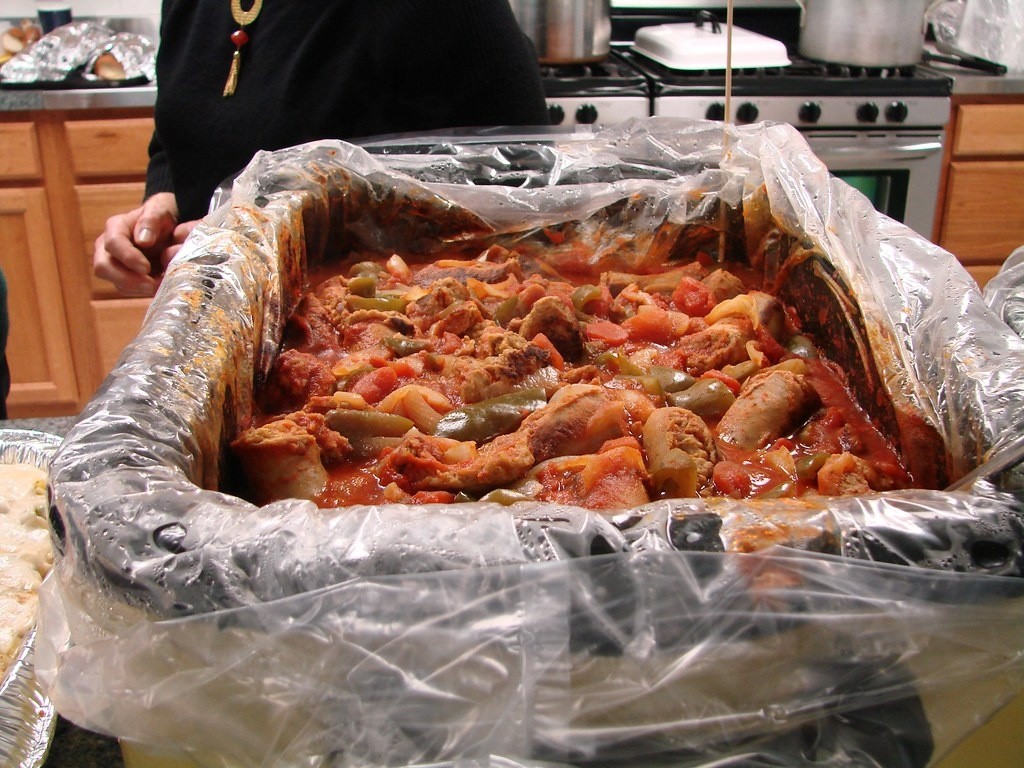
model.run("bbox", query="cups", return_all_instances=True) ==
[38,6,73,35]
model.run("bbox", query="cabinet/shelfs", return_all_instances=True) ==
[0,117,158,418]
[932,94,1024,295]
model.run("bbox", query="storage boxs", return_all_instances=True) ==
[54,142,1024,768]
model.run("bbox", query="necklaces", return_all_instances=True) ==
[223,0,262,98]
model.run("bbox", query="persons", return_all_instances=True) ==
[94,0,549,299]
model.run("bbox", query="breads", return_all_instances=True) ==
[93,51,124,80]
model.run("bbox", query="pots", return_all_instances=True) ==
[796,0,940,66]
[510,0,614,63]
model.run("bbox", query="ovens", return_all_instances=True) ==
[795,128,949,257]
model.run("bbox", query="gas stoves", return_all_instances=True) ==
[540,42,952,128]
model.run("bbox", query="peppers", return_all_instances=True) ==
[325,263,828,505]
